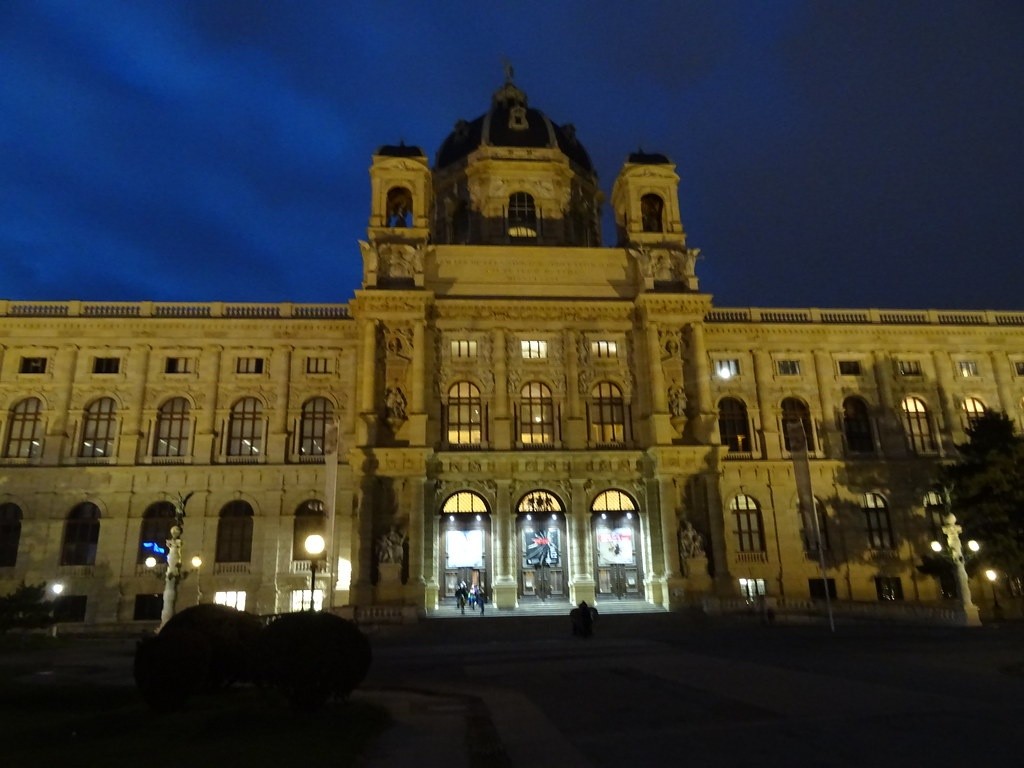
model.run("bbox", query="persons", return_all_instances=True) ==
[679,520,706,559]
[454,581,484,616]
[667,374,688,416]
[384,387,404,418]
[375,526,407,564]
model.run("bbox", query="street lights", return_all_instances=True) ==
[927,512,982,624]
[302,532,327,612]
[143,526,204,637]
